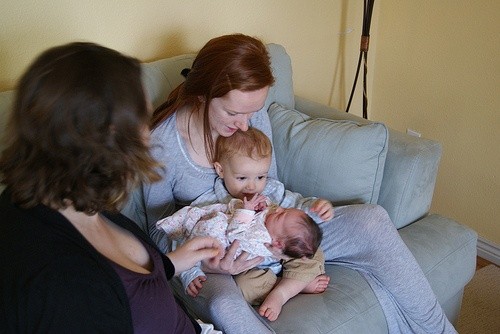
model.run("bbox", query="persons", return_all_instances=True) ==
[115,34,457,334]
[171,125,334,323]
[156,193,323,259]
[0,41,225,333]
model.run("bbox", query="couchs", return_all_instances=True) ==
[0,44,477,334]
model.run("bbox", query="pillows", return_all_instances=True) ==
[267,103,390,205]
